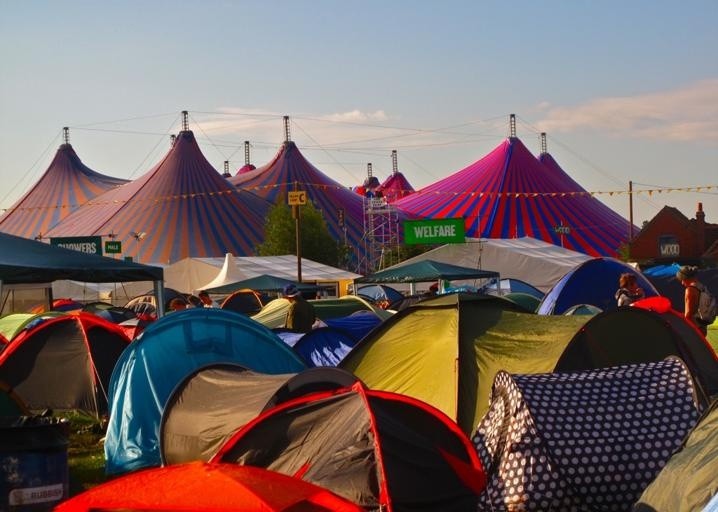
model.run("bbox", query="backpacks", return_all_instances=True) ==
[692,284,718,324]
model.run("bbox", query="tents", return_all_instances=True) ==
[0,235,718,512]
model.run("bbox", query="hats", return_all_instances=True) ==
[284,284,300,298]
[677,266,698,279]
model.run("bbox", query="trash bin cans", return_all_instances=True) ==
[0,415,69,512]
